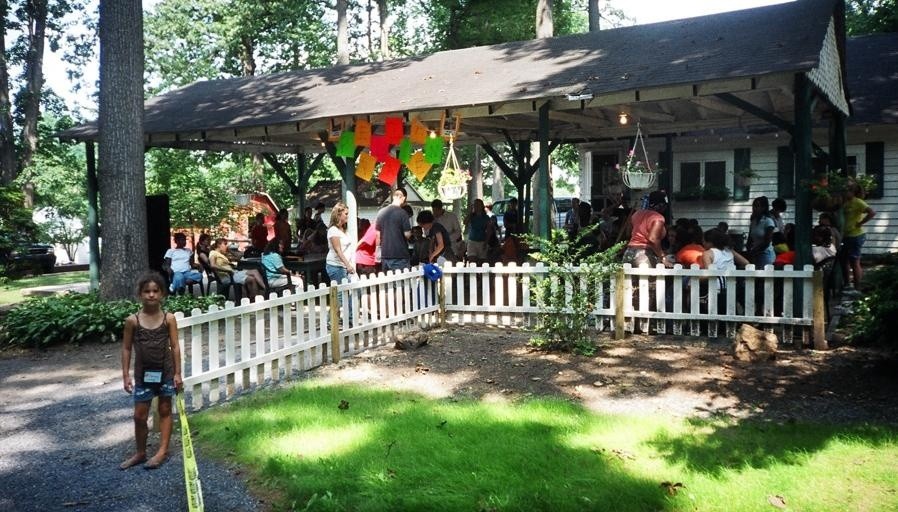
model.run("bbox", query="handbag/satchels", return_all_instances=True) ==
[143,369,163,384]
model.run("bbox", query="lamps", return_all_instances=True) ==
[619,111,628,125]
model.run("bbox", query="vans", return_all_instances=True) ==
[491,197,573,233]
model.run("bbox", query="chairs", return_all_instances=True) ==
[256,260,307,310]
[166,261,205,298]
[209,266,243,300]
[197,256,217,296]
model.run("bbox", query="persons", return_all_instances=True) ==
[120,272,182,470]
[840,183,875,290]
[563,197,842,334]
[162,188,524,315]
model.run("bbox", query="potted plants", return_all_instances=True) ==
[675,184,701,200]
[702,185,731,199]
[11,207,56,274]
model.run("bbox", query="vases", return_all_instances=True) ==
[819,198,834,209]
[735,188,743,200]
[625,173,653,189]
[855,192,868,199]
[443,185,463,197]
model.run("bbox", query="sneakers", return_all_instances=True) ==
[168,283,175,294]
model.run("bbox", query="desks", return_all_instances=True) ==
[238,253,331,305]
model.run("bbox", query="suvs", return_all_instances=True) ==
[0,233,56,274]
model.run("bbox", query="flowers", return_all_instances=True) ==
[436,168,473,185]
[729,167,761,187]
[615,149,664,176]
[843,172,879,194]
[798,169,848,197]
